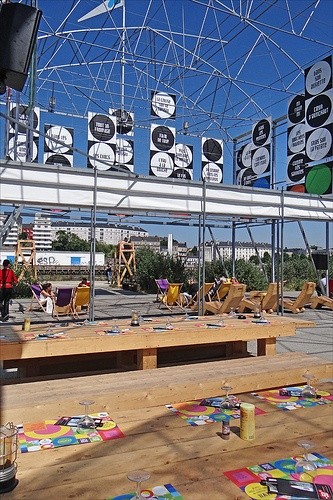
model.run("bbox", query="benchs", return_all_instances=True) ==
[0,352,333,429]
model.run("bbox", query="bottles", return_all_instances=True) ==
[222,420,230,440]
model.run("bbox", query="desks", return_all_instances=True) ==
[0,313,296,370]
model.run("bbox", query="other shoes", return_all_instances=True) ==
[0,317,9,321]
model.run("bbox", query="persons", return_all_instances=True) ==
[230,277,240,285]
[0,259,17,321]
[78,278,90,311]
[38,283,58,316]
[316,273,327,297]
[201,274,227,302]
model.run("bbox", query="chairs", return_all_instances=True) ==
[156,279,333,314]
[27,283,90,321]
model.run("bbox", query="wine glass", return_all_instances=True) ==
[300,374,318,401]
[184,307,266,326]
[138,312,144,323]
[76,399,96,435]
[294,439,317,482]
[46,323,53,337]
[166,317,172,329]
[111,319,120,333]
[126,470,152,500]
[83,316,90,327]
[220,387,233,415]
